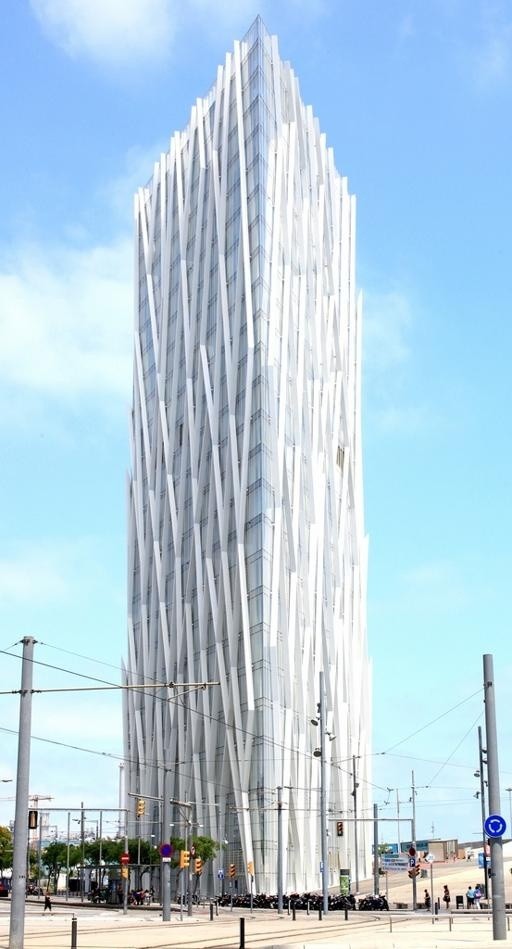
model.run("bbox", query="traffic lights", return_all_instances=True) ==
[229,863,235,877]
[195,858,202,876]
[180,850,190,869]
[137,800,145,817]
[337,822,343,836]
[247,862,253,873]
[121,868,128,879]
[408,864,420,879]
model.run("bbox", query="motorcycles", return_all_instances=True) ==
[213,892,390,911]
[176,892,201,905]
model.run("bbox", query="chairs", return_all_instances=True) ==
[456,896,464,909]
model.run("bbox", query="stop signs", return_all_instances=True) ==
[119,853,130,864]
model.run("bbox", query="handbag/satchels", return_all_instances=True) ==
[475,893,481,897]
[444,896,450,901]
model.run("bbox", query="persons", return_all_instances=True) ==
[131,885,154,905]
[466,886,474,909]
[444,885,450,908]
[424,889,431,911]
[474,886,481,909]
[42,892,54,916]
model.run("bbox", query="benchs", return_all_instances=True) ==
[416,903,427,909]
[393,903,409,909]
